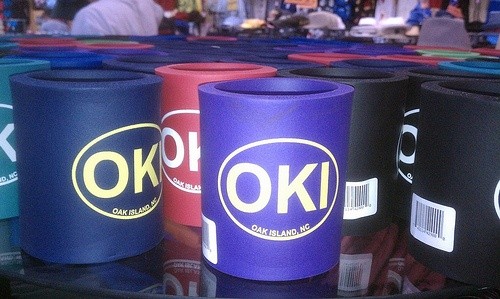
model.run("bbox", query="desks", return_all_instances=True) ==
[0,214,498,299]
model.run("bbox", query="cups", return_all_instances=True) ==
[0,33,499,283]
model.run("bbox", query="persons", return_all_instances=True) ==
[41,1,90,34]
[406,0,432,25]
[73,0,164,36]
